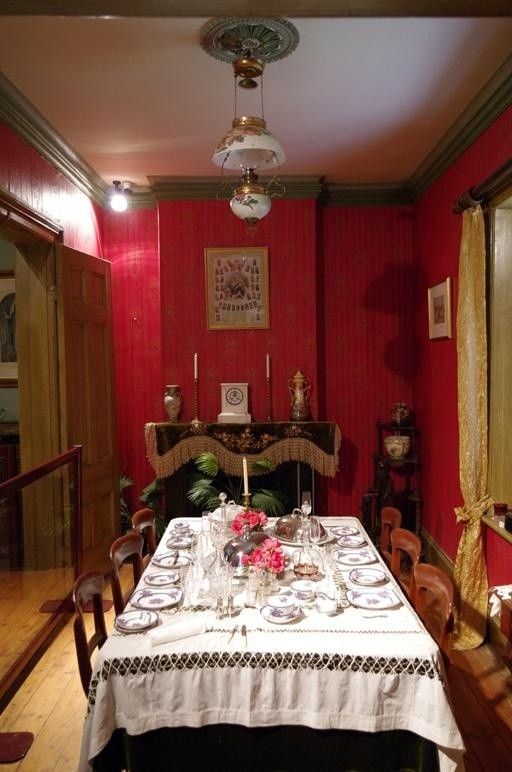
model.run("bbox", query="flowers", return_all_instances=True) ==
[277,526,290,541]
[231,511,268,535]
[239,538,289,578]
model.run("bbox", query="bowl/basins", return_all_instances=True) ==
[212,519,233,528]
[386,436,410,460]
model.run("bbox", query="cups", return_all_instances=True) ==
[266,595,295,617]
[201,511,212,532]
[291,580,316,602]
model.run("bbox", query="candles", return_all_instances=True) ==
[242,456,250,494]
[193,352,199,379]
[266,353,271,378]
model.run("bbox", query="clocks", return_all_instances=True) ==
[217,382,253,424]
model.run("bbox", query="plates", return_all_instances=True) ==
[265,525,334,545]
[259,606,305,623]
[330,525,400,611]
[116,521,205,632]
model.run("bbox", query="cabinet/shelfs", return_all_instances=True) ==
[362,417,424,559]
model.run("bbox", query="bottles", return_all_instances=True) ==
[292,526,324,579]
[228,594,235,617]
[216,594,224,619]
[206,544,234,592]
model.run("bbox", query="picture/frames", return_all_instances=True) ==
[427,276,454,341]
[0,269,19,388]
[203,245,273,331]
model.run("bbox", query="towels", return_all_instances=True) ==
[148,613,207,647]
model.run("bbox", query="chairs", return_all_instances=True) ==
[106,531,144,615]
[407,562,456,676]
[131,507,157,562]
[379,507,402,566]
[69,570,109,699]
[388,527,422,602]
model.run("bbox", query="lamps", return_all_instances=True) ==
[198,16,302,239]
